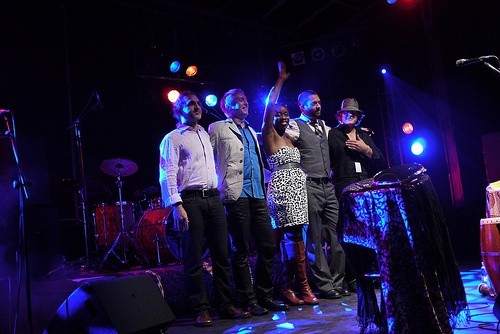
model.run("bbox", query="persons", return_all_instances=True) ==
[261,60,317,304]
[160,88,388,324]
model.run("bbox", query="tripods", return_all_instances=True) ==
[97,170,153,274]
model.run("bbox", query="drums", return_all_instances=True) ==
[93,199,137,250]
[479,217,500,294]
[144,196,165,209]
[134,209,211,263]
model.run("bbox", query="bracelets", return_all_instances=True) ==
[174,202,181,206]
[366,146,371,154]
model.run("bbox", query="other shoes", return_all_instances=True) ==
[349,279,357,292]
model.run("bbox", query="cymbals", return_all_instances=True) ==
[100,158,138,177]
[121,185,161,201]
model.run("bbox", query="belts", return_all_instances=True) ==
[306,177,331,182]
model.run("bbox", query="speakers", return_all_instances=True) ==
[42,273,176,334]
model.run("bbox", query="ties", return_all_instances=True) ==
[314,123,324,140]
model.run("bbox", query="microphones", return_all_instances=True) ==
[96,93,102,111]
[456,56,497,66]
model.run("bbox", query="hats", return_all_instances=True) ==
[337,97,363,115]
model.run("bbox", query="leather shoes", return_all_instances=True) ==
[265,302,289,312]
[320,288,351,299]
[195,310,213,327]
[243,304,269,316]
[181,188,221,197]
[221,306,251,320]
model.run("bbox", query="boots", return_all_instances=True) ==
[279,240,305,306]
[293,239,320,305]
